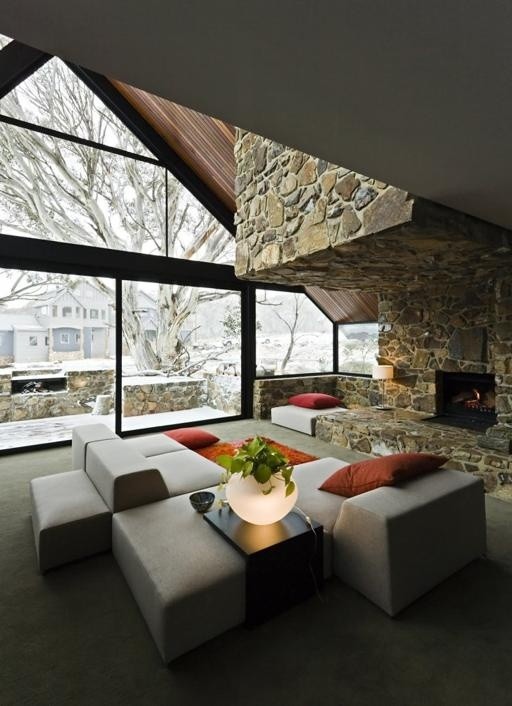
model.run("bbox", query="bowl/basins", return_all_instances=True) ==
[189,491,215,513]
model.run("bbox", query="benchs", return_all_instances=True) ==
[112,458,485,666]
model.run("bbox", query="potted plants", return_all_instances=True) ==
[223,437,297,524]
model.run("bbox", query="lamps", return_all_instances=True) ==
[373,366,394,411]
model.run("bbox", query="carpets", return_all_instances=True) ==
[191,436,318,466]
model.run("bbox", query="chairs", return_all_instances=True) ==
[29,425,232,577]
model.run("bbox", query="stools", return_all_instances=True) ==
[270,405,349,437]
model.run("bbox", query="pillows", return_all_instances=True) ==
[319,453,451,497]
[287,393,342,409]
